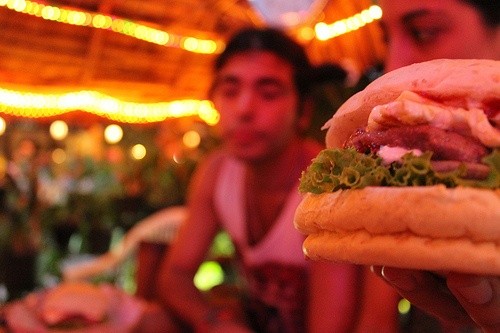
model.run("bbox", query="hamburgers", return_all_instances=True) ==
[290,58,499,278]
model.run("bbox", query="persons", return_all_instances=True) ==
[0,122,221,301]
[305,57,384,147]
[153,25,364,330]
[379,0,499,331]
